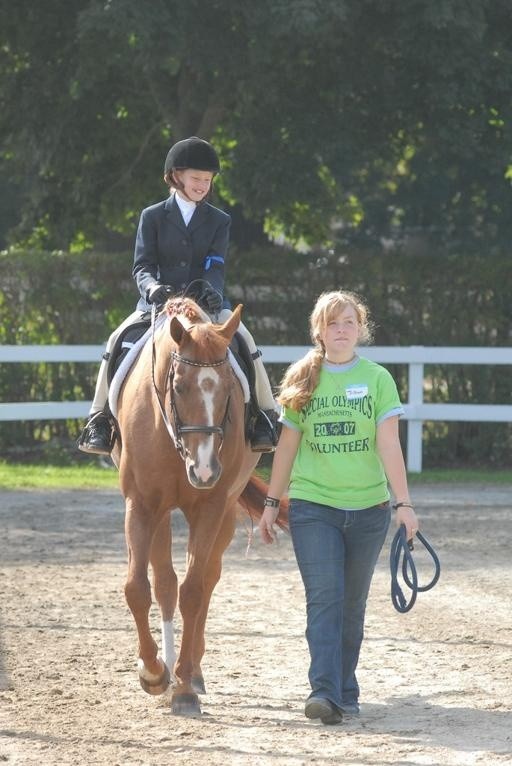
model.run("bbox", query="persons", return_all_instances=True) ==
[257,288,419,726]
[76,135,282,456]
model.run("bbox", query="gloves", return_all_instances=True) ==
[204,287,224,313]
[146,283,173,306]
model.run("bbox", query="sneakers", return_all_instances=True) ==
[75,412,112,455]
[304,695,344,726]
[249,409,282,453]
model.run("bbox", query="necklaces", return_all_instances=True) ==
[323,352,357,365]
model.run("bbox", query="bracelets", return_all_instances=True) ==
[263,495,280,509]
[393,501,414,510]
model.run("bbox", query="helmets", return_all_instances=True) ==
[163,136,222,179]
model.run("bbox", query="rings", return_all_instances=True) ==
[410,527,416,534]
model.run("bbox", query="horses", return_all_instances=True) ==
[108,296,292,722]
